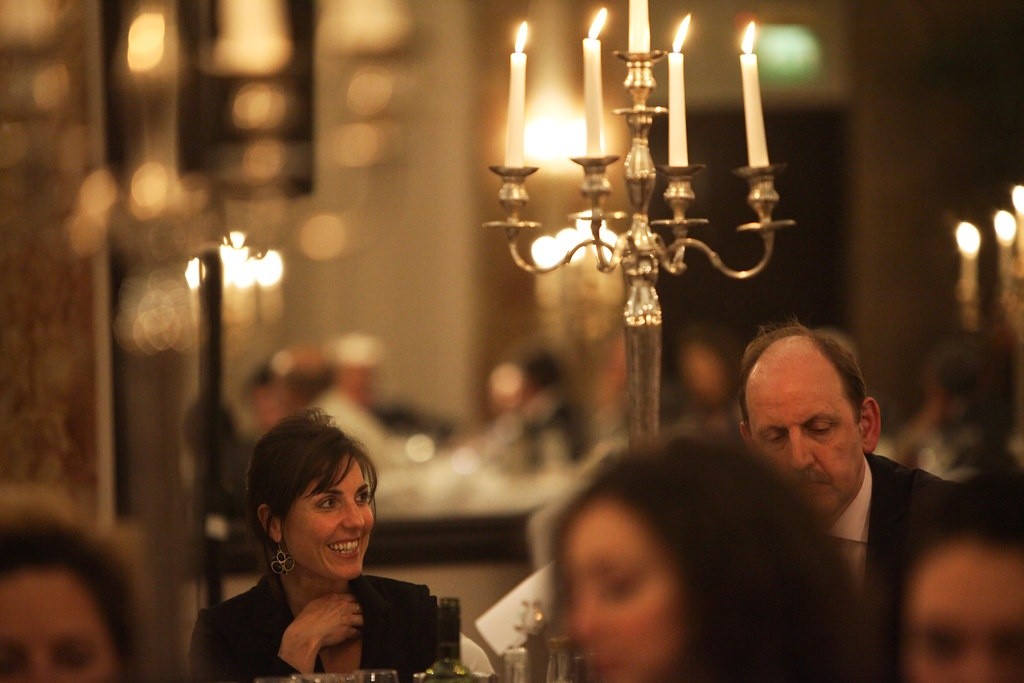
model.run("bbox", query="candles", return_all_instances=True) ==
[739,20,769,166]
[581,7,611,157]
[953,219,983,335]
[667,13,693,167]
[628,0,650,55]
[501,21,530,169]
[992,208,1018,321]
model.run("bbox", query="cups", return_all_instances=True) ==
[254,669,398,683]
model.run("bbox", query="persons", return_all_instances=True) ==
[541,428,860,683]
[872,467,1023,683]
[181,408,447,683]
[738,323,970,597]
[222,321,1024,488]
[0,475,162,683]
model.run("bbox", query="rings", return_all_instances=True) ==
[352,601,362,615]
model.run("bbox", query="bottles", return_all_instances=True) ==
[502,646,532,683]
[545,636,574,683]
[422,598,479,683]
